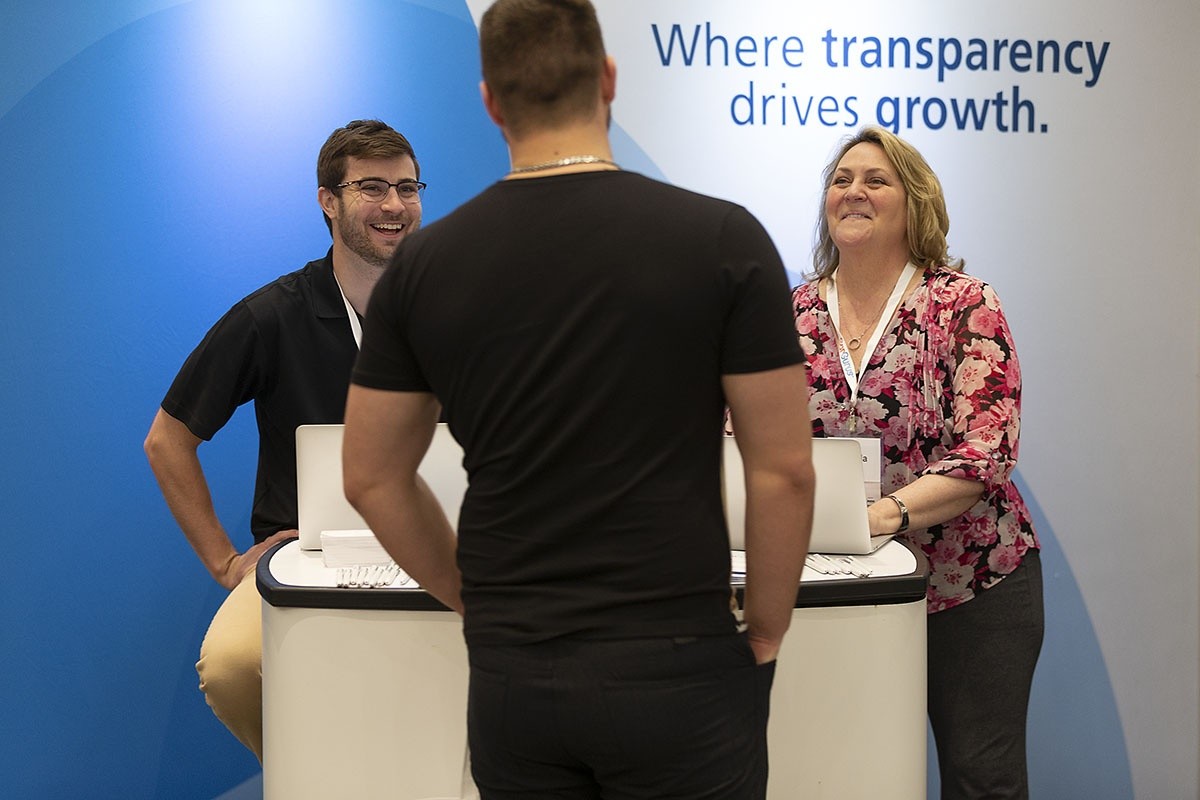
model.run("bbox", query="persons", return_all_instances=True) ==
[141,121,428,774]
[784,127,1044,800]
[339,1,792,800]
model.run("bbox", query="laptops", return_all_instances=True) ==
[295,424,469,551]
[722,435,893,554]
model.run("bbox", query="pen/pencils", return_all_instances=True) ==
[841,555,873,579]
[335,560,411,588]
[804,554,851,574]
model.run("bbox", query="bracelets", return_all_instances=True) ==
[883,493,910,535]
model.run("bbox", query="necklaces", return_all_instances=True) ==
[507,156,620,175]
[838,257,909,350]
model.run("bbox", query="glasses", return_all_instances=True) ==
[336,178,426,204]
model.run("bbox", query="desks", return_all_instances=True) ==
[254,535,930,799]
